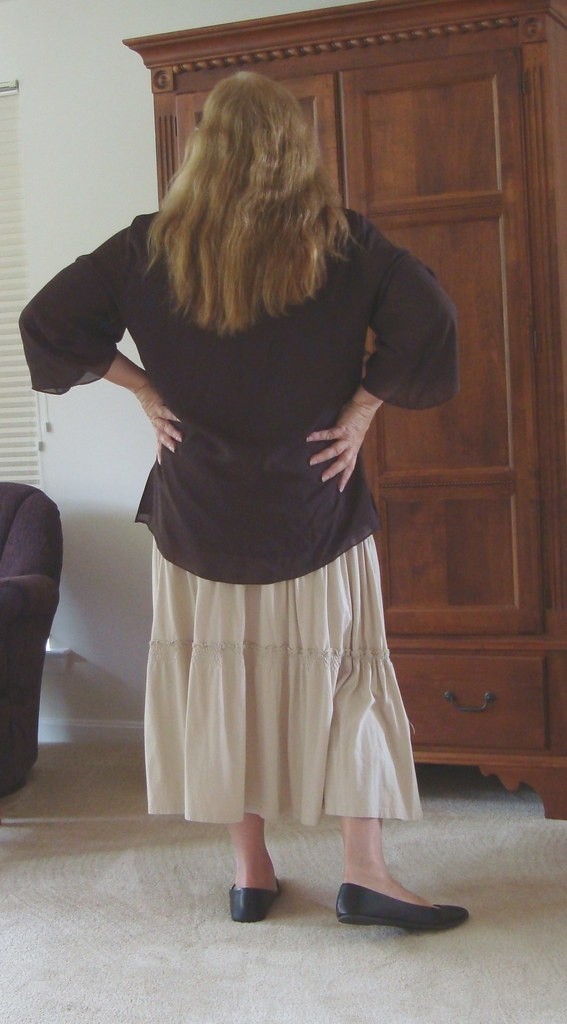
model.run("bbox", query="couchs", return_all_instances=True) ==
[0,481,65,797]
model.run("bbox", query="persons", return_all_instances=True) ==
[20,70,470,935]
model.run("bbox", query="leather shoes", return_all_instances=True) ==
[336,883,468,929]
[230,876,281,923]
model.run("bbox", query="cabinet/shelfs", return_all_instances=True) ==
[121,0,567,821]
